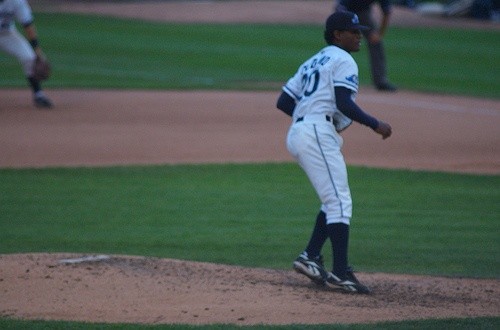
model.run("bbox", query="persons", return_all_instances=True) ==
[275,9,391,292]
[332,0,398,92]
[0,0,53,108]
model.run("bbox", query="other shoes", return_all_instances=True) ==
[378,81,397,91]
[33,95,53,109]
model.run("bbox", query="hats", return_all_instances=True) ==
[325,10,372,32]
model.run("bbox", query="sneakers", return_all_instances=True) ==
[320,267,370,294]
[293,251,328,281]
